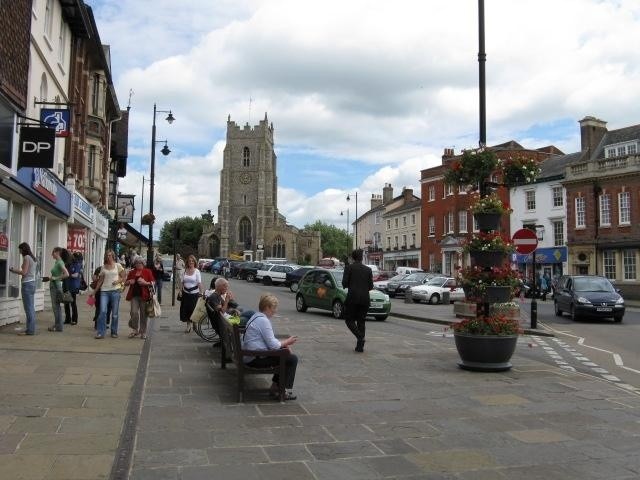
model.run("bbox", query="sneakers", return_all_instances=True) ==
[95,334,104,339]
[140,332,146,339]
[17,329,34,335]
[111,334,118,338]
[48,325,64,332]
[127,329,139,338]
[355,340,365,352]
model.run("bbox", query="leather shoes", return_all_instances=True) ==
[269,388,297,400]
[287,391,292,394]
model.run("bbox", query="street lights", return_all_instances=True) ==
[340,209,349,256]
[346,192,358,250]
[146,102,176,268]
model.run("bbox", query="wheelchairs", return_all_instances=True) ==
[191,294,220,342]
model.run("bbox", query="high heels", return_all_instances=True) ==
[64,317,71,324]
[71,314,78,325]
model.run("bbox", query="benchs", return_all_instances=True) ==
[204,302,246,348]
[219,311,291,403]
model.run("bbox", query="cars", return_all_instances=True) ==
[160,258,174,280]
[409,277,466,304]
[285,266,323,294]
[197,256,296,284]
[293,263,391,322]
[373,273,403,291]
[554,275,625,322]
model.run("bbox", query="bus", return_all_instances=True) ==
[319,258,340,268]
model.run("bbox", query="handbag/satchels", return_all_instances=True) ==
[55,289,74,304]
[78,277,88,291]
[177,293,183,301]
[7,284,19,298]
[146,297,155,318]
[119,282,125,293]
[152,294,162,319]
[90,281,97,289]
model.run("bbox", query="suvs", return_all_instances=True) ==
[387,272,440,297]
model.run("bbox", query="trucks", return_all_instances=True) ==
[396,267,425,275]
[366,264,378,272]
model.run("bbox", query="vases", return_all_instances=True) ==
[463,286,477,299]
[486,286,512,305]
[476,214,500,230]
[474,252,504,267]
[508,169,526,183]
[454,335,519,371]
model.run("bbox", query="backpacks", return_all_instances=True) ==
[190,296,208,325]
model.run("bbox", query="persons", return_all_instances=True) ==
[63,248,82,326]
[513,268,559,301]
[175,253,184,290]
[240,291,299,401]
[341,249,374,352]
[203,276,218,299]
[94,248,126,338]
[9,241,39,337]
[178,254,203,334]
[47,246,69,332]
[91,265,112,330]
[220,258,231,275]
[125,256,157,340]
[207,278,242,316]
[153,252,164,306]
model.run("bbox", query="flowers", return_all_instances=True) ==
[444,141,501,190]
[486,264,524,287]
[467,192,513,216]
[458,231,518,255]
[501,156,542,185]
[444,314,524,336]
[454,265,485,303]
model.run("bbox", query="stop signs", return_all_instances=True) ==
[511,228,538,255]
